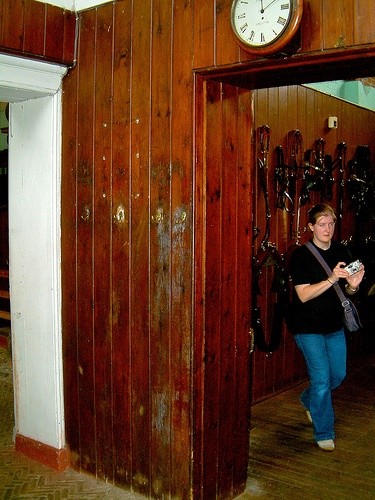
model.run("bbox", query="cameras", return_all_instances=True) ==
[344,259,361,276]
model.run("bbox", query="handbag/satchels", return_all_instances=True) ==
[342,299,362,332]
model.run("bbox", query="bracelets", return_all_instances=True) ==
[346,285,359,295]
[326,279,334,285]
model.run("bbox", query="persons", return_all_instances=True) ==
[292,203,365,452]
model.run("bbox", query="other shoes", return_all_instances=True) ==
[306,408,312,422]
[318,439,334,451]
